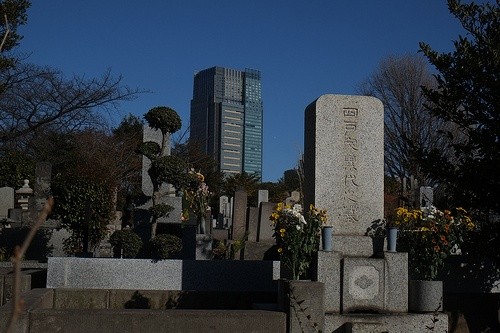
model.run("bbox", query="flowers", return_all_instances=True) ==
[385,207,476,281]
[271,202,329,280]
[179,167,213,221]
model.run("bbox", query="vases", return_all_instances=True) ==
[200,216,206,234]
[409,280,444,312]
